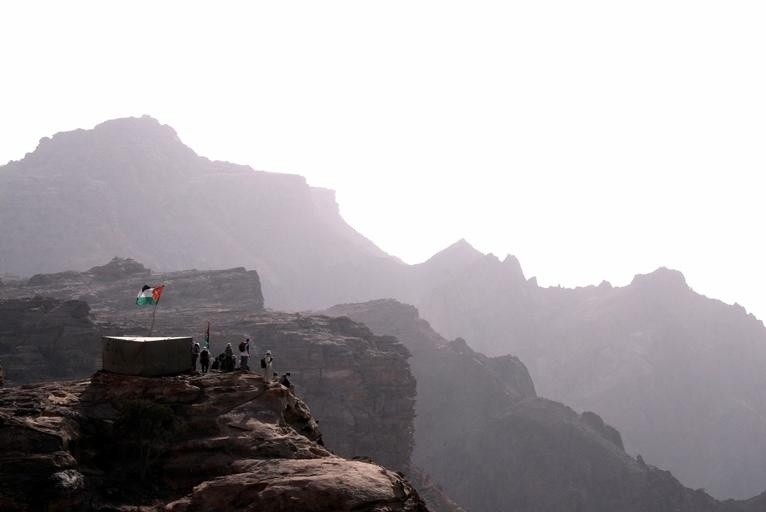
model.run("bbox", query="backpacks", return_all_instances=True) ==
[239,341,247,352]
[260,358,267,368]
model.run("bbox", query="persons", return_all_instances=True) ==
[261,350,273,384]
[279,370,295,394]
[192,339,252,373]
[273,372,282,385]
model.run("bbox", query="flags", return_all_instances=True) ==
[134,285,163,306]
[203,323,210,348]
